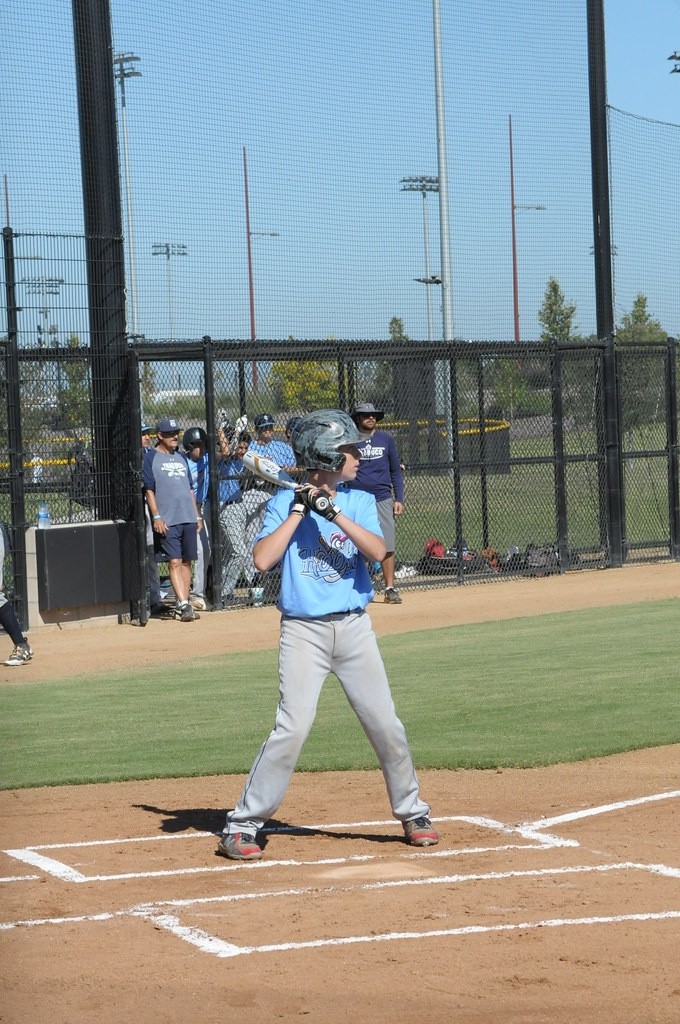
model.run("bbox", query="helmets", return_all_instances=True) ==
[285,416,301,430]
[291,409,375,473]
[229,431,252,444]
[254,414,275,427]
[216,426,235,444]
[183,427,206,451]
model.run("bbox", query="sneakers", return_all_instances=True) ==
[402,817,438,846]
[173,602,200,622]
[218,832,263,860]
[4,636,33,666]
[384,587,402,604]
[394,565,418,579]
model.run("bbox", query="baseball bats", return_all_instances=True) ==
[242,450,329,512]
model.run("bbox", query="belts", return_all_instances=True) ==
[225,499,243,505]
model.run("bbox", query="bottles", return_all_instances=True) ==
[38,504,50,529]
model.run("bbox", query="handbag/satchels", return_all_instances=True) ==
[415,539,580,574]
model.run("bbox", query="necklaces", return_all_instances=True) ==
[356,434,374,462]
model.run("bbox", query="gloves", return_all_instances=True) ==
[300,486,342,523]
[291,484,310,519]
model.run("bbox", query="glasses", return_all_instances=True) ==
[163,430,181,434]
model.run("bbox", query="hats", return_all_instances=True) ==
[348,403,385,422]
[141,421,155,432]
[156,418,185,432]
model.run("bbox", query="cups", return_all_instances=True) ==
[252,588,264,606]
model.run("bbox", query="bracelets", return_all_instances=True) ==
[153,515,160,522]
[197,517,202,520]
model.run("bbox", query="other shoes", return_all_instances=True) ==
[151,604,174,618]
[189,595,213,610]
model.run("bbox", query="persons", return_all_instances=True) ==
[216,414,308,610]
[30,453,43,484]
[180,408,230,610]
[0,523,34,666]
[140,418,171,618]
[143,419,203,622]
[218,408,440,861]
[346,403,404,604]
